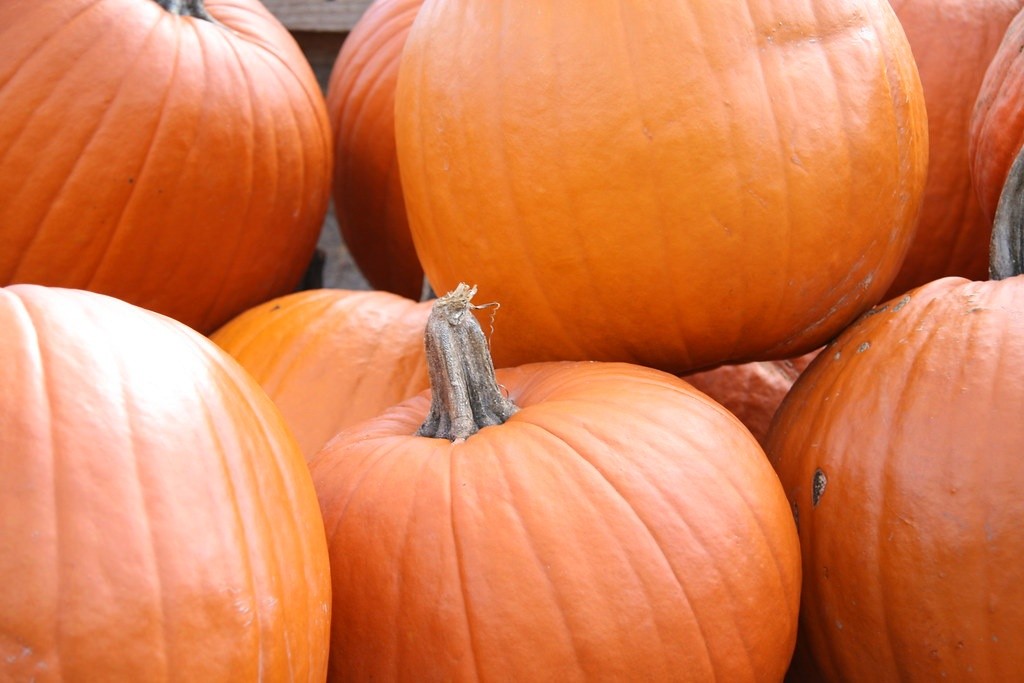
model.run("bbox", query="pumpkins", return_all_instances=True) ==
[0,0,1024,683]
